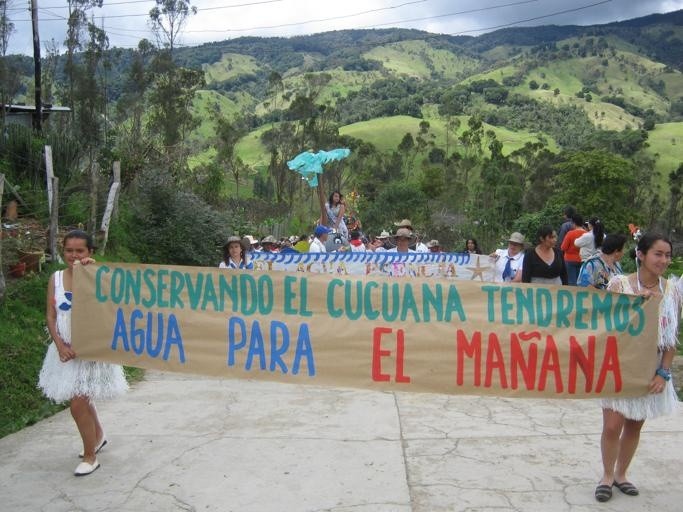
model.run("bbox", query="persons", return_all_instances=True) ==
[561,214,588,285]
[575,217,610,267]
[490,231,526,284]
[596,234,680,500]
[324,191,349,239]
[460,238,483,254]
[521,225,571,285]
[218,236,252,271]
[37,231,130,476]
[577,230,629,292]
[244,217,445,253]
[558,206,576,268]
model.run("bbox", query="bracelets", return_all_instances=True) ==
[658,369,670,379]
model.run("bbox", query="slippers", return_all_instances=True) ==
[595,478,615,501]
[615,480,638,495]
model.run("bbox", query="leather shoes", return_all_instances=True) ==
[74,457,100,476]
[78,435,107,458]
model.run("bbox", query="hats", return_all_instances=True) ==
[224,235,280,253]
[315,219,440,248]
[505,232,533,250]
